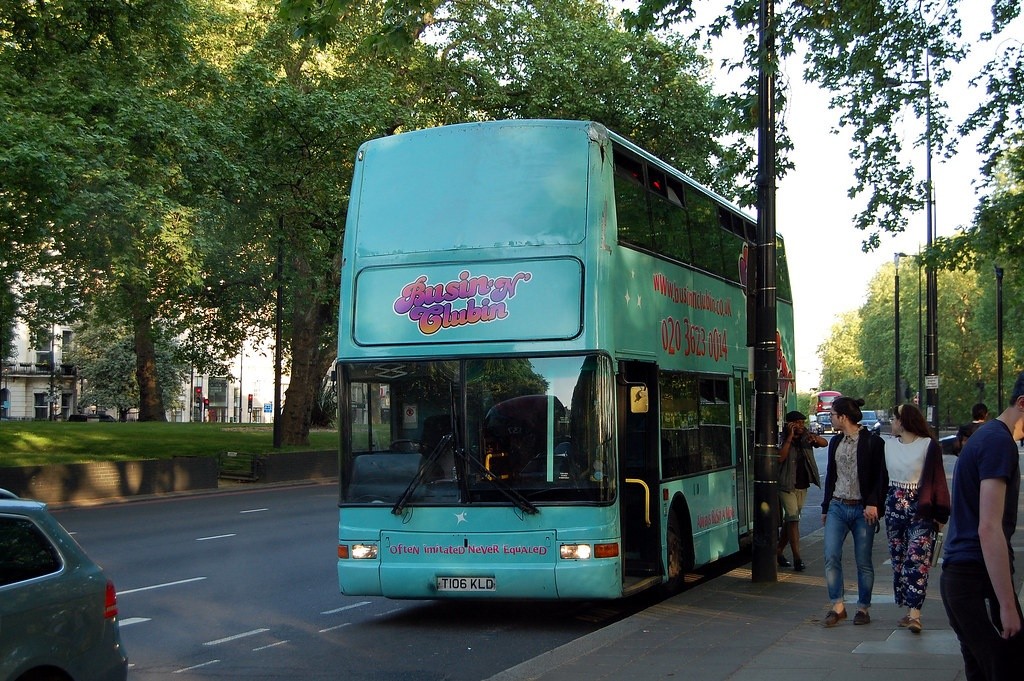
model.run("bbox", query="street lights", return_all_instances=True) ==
[992,258,1003,416]
[892,252,909,408]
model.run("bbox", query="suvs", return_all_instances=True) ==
[0,488,129,681]
[816,411,839,435]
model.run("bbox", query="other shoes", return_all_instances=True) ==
[794,559,805,570]
[777,555,791,567]
[908,618,922,633]
[854,610,870,624]
[897,615,909,627]
[821,607,848,625]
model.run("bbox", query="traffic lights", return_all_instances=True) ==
[194,387,202,403]
[248,394,253,413]
[205,399,209,409]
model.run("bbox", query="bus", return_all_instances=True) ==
[337,119,798,601]
[808,390,842,433]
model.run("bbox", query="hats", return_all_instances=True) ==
[786,410,806,421]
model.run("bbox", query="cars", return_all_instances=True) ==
[90,414,115,422]
[857,410,881,435]
[808,415,821,435]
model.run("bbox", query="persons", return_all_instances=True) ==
[939,372,1024,681]
[821,397,886,627]
[956,403,988,453]
[863,405,949,633]
[779,411,828,570]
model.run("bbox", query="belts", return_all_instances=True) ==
[833,496,857,505]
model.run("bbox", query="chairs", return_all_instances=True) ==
[419,415,463,459]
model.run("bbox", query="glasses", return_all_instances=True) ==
[829,413,841,418]
[889,416,897,424]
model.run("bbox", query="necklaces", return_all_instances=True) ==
[912,434,917,442]
